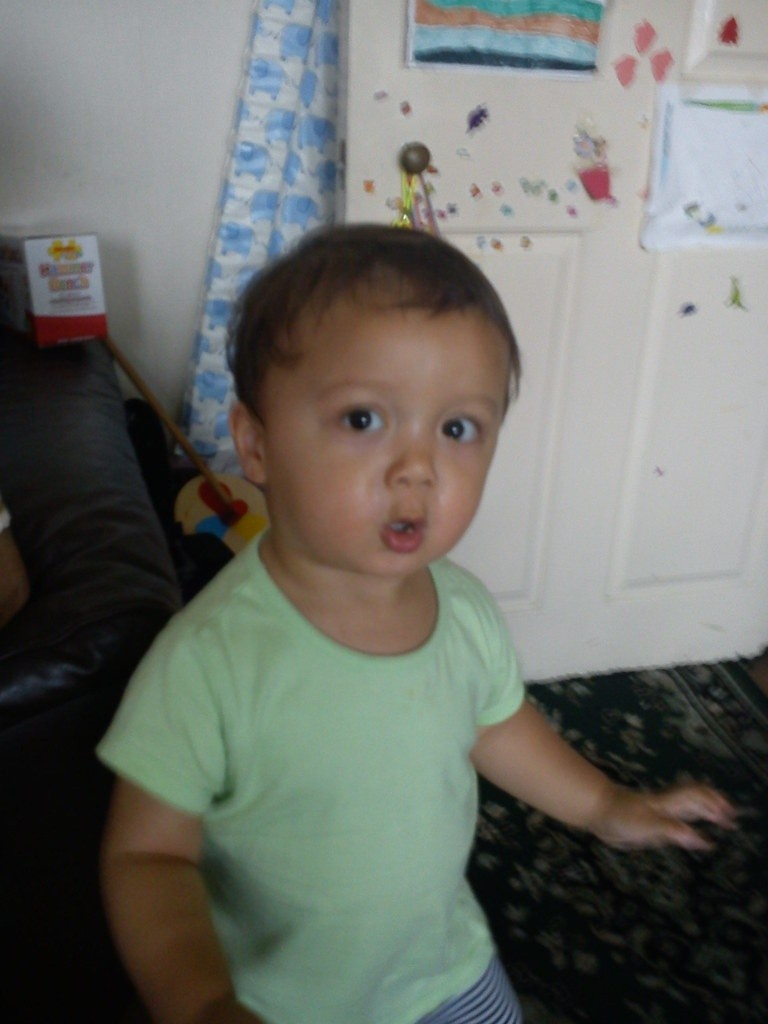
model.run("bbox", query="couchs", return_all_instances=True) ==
[0,328,193,1024]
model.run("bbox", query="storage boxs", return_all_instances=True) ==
[23,236,110,346]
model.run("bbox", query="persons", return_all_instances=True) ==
[97,222,737,1023]
